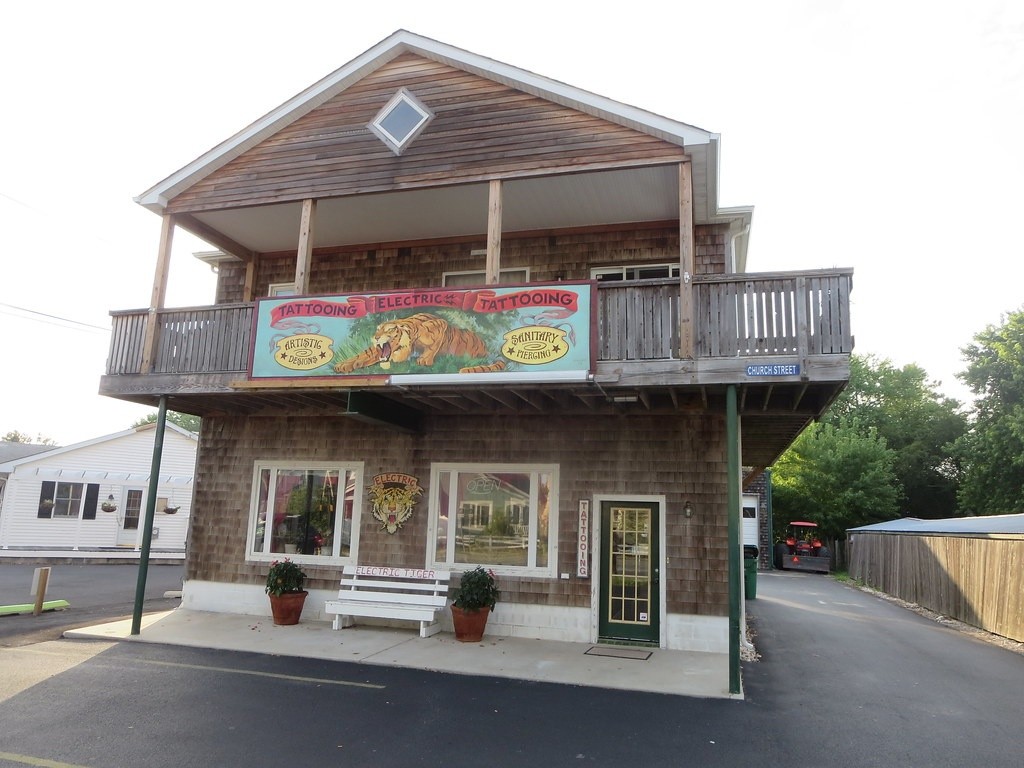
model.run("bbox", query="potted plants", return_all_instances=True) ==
[39,498,54,510]
[101,502,116,512]
[163,506,181,514]
[264,557,308,625]
[449,565,502,642]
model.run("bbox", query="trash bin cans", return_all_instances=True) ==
[744,543,760,599]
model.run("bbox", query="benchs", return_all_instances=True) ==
[324,565,451,638]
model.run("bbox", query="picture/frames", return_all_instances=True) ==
[246,279,598,380]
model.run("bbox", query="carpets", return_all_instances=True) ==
[583,646,653,660]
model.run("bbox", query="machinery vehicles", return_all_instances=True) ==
[774,522,831,575]
